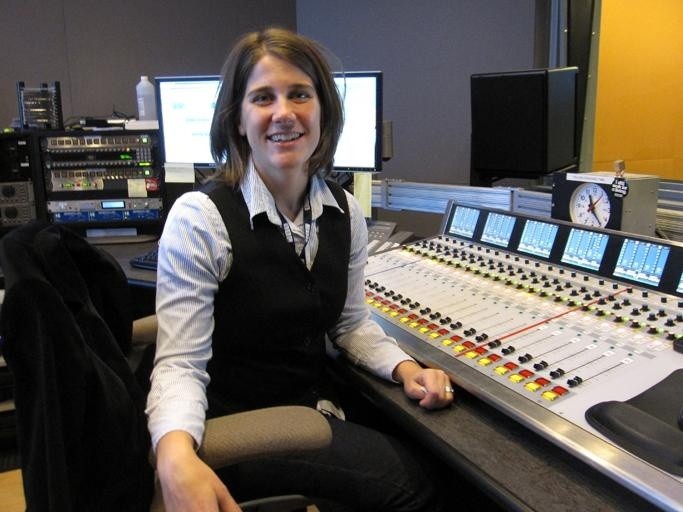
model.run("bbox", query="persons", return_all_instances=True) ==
[141,24,456,511]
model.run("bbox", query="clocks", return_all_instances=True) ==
[550,171,658,238]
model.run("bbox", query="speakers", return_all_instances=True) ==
[469,65,580,186]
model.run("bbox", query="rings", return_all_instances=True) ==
[446,387,455,393]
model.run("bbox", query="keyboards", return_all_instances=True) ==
[129,247,158,270]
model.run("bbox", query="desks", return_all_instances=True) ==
[93,238,683,512]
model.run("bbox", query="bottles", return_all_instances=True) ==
[137,76,157,121]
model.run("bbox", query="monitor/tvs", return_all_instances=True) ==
[153,70,386,176]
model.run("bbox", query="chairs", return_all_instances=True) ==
[1,217,334,512]
